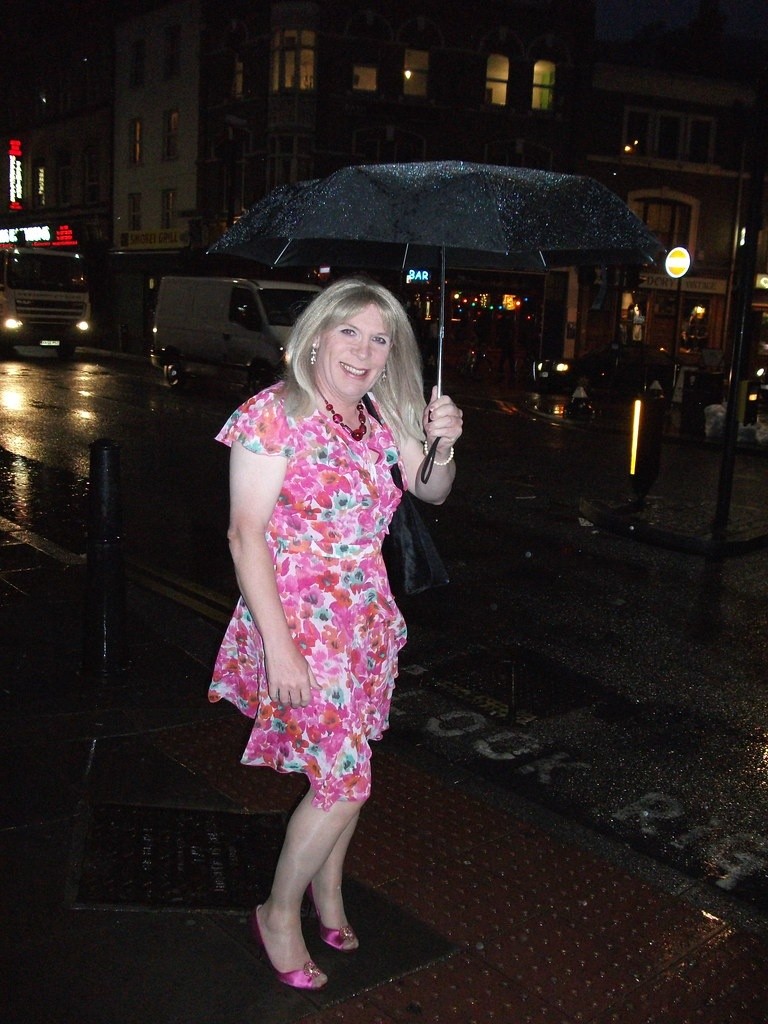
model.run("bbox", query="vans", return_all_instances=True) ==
[150,275,327,390]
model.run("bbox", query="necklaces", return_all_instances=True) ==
[311,379,367,442]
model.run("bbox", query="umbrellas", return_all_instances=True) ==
[202,164,663,489]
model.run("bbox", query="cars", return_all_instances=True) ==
[532,345,662,393]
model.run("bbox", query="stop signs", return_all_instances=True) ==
[664,247,691,279]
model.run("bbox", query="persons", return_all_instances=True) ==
[204,277,464,992]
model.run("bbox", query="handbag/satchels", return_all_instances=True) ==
[362,394,450,604]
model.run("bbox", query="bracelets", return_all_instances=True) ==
[423,441,455,466]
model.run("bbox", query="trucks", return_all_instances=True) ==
[0,246,91,352]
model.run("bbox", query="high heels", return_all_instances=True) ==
[248,906,328,991]
[301,882,360,954]
